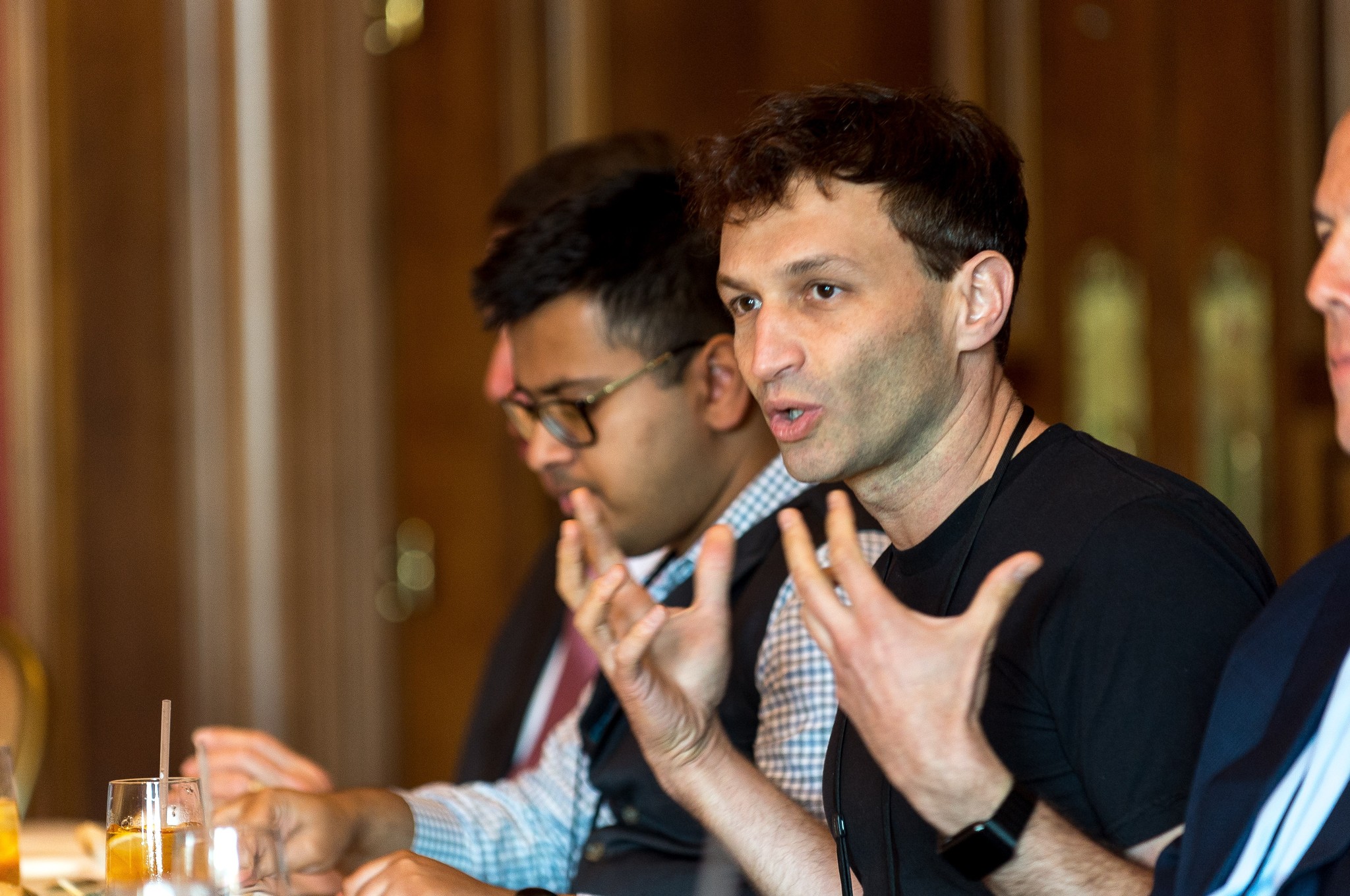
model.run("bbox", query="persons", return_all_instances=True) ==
[552,72,1277,896]
[190,115,893,896]
[159,128,695,827]
[1145,103,1350,896]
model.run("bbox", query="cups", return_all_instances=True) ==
[106,776,209,896]
[1,745,21,896]
[173,826,291,895]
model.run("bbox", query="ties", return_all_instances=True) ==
[511,605,597,781]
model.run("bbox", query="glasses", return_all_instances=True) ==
[497,339,707,450]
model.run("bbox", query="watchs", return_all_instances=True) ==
[939,774,1040,884]
